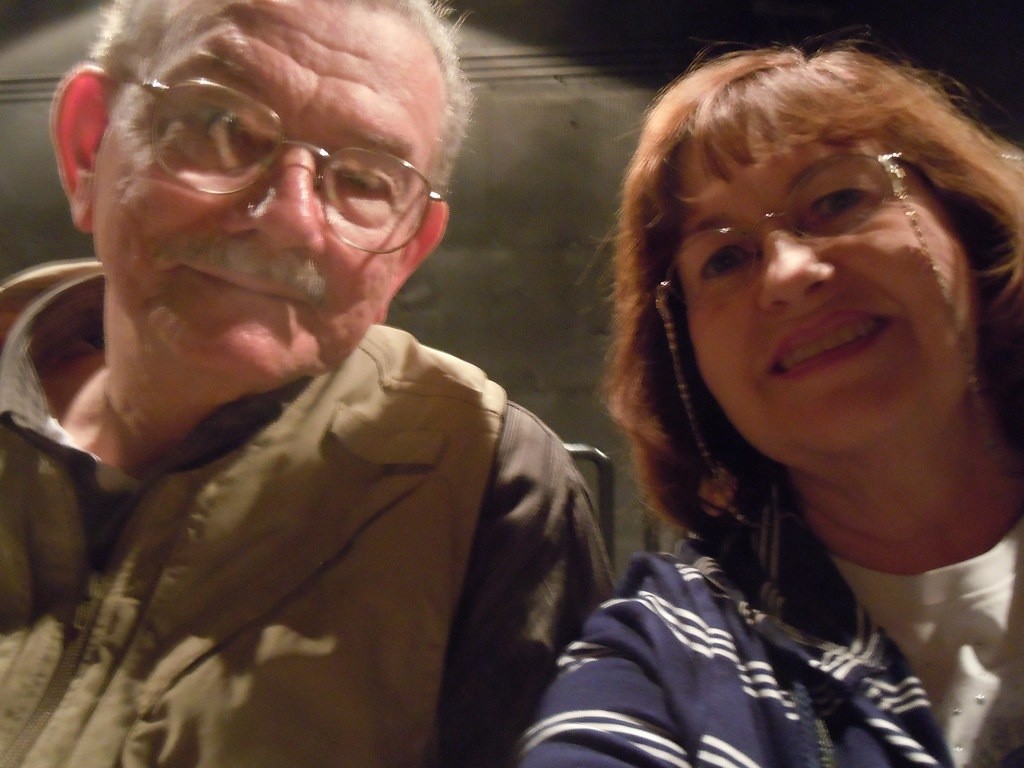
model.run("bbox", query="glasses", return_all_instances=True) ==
[126,73,451,253]
[657,154,904,304]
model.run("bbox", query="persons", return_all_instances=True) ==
[521,43,1024,768]
[0,0,616,768]
